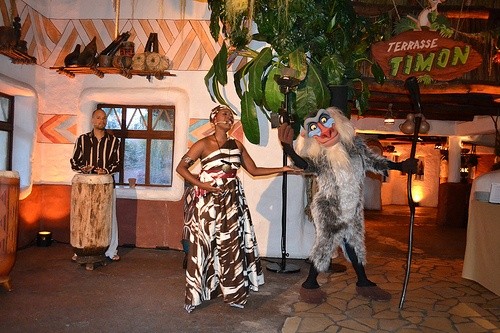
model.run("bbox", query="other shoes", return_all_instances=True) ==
[72,253,77,260]
[112,255,120,261]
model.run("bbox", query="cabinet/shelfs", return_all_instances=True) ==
[0,171,20,293]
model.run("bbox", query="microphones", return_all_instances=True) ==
[217,121,227,124]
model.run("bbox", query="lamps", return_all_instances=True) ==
[384,103,395,125]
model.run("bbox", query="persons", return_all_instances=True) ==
[175,104,304,314]
[69,110,121,262]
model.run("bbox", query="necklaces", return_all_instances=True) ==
[213,132,231,174]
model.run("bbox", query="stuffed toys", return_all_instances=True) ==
[277,106,425,304]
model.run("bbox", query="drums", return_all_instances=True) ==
[70,174,116,270]
[0,169,22,291]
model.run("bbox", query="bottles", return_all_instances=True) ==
[419,161,423,175]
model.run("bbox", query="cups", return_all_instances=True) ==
[128,178,136,188]
